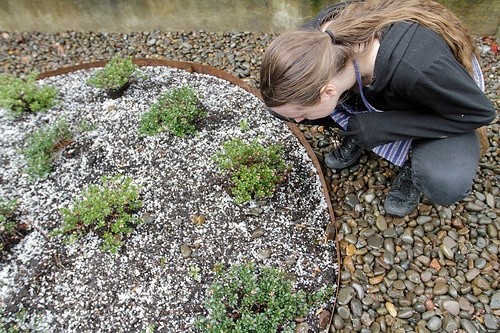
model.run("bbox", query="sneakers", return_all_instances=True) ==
[384,156,421,218]
[325,135,365,169]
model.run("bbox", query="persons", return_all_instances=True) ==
[260,0,497,217]
